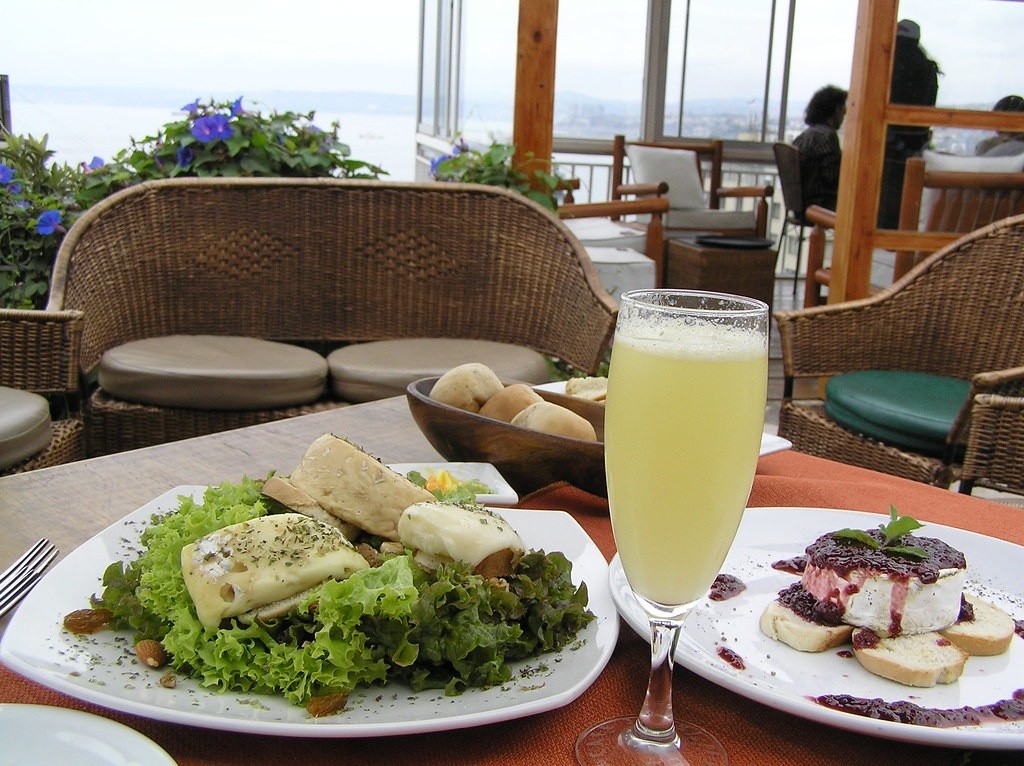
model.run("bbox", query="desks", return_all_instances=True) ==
[1,372,1024,766]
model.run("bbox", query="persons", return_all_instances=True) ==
[792,86,847,210]
[878,20,938,230]
[982,96,1024,156]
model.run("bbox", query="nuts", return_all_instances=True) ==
[134,640,167,668]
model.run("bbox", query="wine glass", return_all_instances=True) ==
[575,289,770,766]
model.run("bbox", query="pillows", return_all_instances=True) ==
[916,150,1024,236]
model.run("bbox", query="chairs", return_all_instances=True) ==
[798,157,1024,309]
[769,207,1024,502]
[0,307,89,479]
[763,144,832,295]
[613,134,771,241]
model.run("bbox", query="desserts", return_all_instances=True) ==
[800,505,968,638]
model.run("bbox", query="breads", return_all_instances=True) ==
[760,591,1015,688]
[430,362,607,442]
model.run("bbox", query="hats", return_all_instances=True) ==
[895,19,920,40]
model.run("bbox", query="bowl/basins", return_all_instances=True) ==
[405,374,607,501]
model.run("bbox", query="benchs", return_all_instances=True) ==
[43,178,616,452]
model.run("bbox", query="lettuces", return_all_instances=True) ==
[90,470,598,706]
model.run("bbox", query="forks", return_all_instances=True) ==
[0,536,60,618]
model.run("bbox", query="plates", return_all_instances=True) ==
[385,461,519,506]
[607,505,1024,751]
[530,379,794,457]
[1,702,177,766]
[0,483,623,740]
